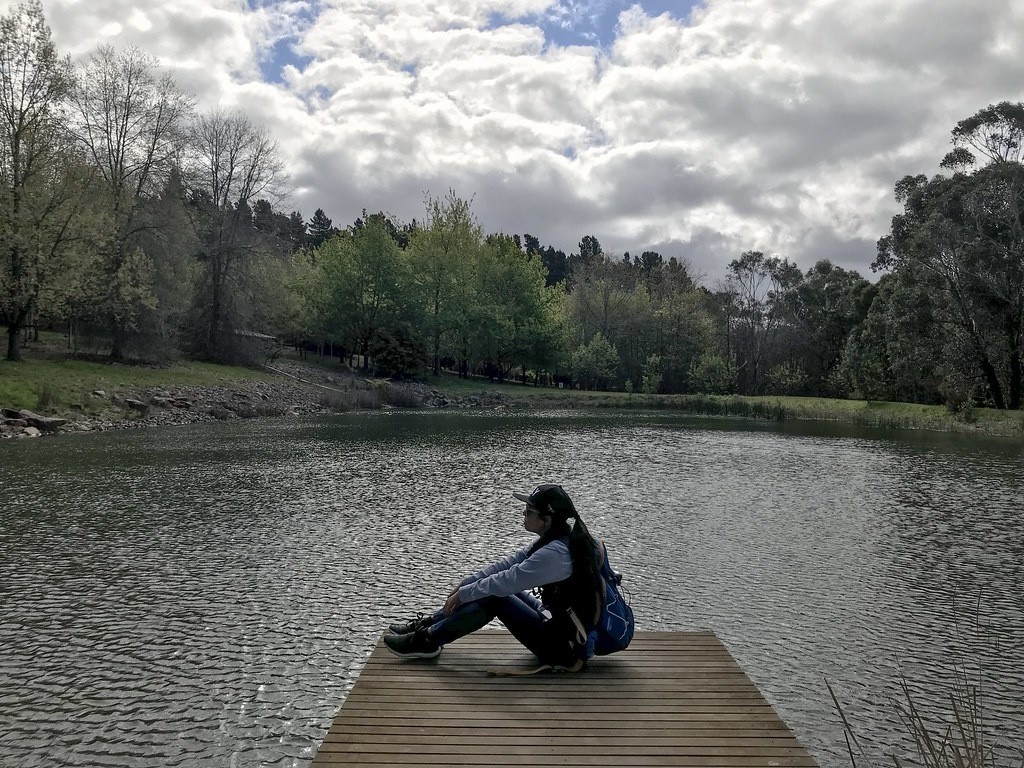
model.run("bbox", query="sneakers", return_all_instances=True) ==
[389,616,435,637]
[384,628,441,657]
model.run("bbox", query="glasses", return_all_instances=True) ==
[524,504,541,520]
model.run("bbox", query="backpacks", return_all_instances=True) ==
[566,539,635,657]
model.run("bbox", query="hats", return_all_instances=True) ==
[512,485,577,517]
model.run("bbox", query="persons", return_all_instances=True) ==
[383,484,602,673]
